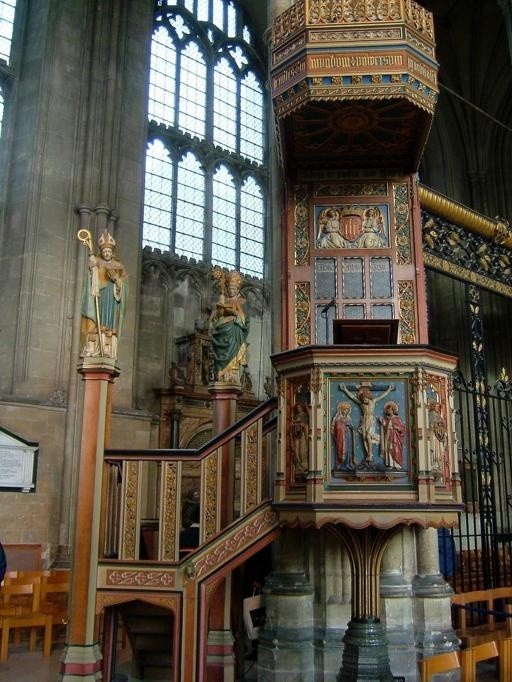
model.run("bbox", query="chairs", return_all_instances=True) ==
[0,570,73,666]
[421,587,512,682]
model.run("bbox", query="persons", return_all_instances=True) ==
[339,382,395,463]
[77,246,130,361]
[207,285,252,387]
[427,400,450,478]
[378,400,406,473]
[315,207,348,250]
[330,401,359,470]
[290,401,311,475]
[354,205,388,248]
[181,487,199,530]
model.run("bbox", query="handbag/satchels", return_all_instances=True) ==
[242,584,266,640]
